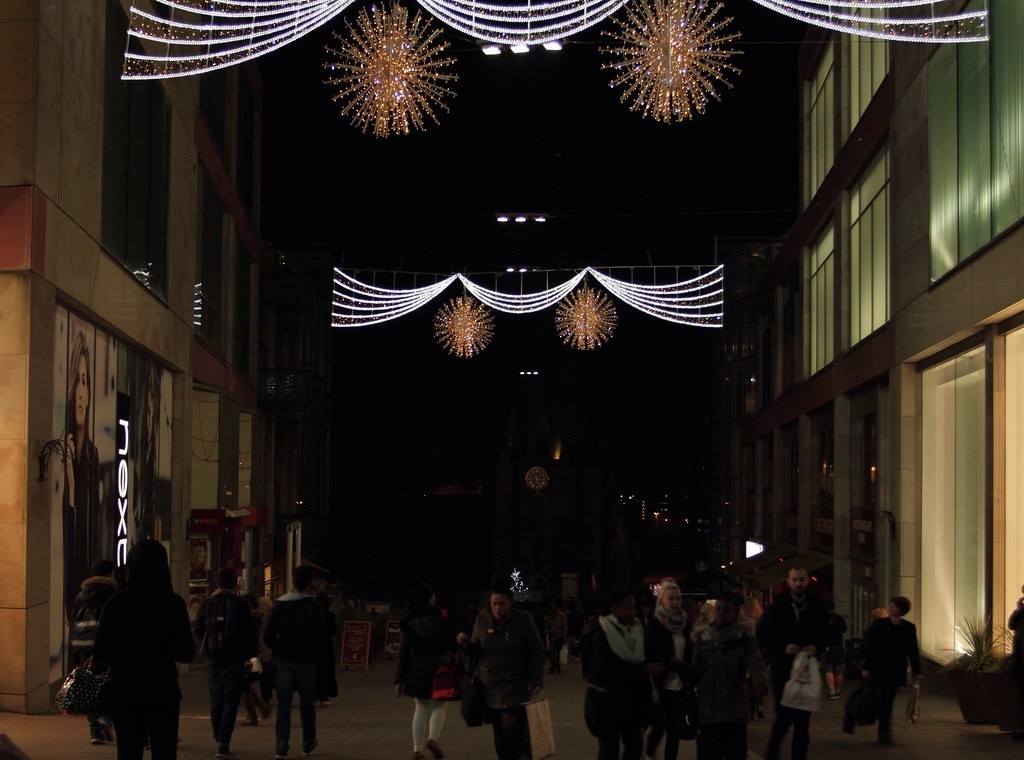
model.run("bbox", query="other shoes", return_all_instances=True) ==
[215,741,236,759]
[427,740,444,760]
[302,741,319,756]
[88,716,114,744]
[412,755,426,760]
[275,753,288,759]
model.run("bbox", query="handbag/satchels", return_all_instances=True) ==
[520,691,557,760]
[778,651,822,714]
[55,655,110,715]
[430,656,463,701]
[559,643,569,665]
[460,646,484,727]
[903,682,922,727]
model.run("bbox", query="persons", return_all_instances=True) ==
[1007,584,1024,670]
[394,566,921,759]
[69,537,336,760]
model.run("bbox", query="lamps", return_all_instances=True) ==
[496,213,550,223]
[477,35,570,56]
[506,266,541,274]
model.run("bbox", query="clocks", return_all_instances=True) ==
[524,468,551,494]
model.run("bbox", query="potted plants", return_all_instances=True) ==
[935,612,1024,731]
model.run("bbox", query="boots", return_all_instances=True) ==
[237,694,272,727]
